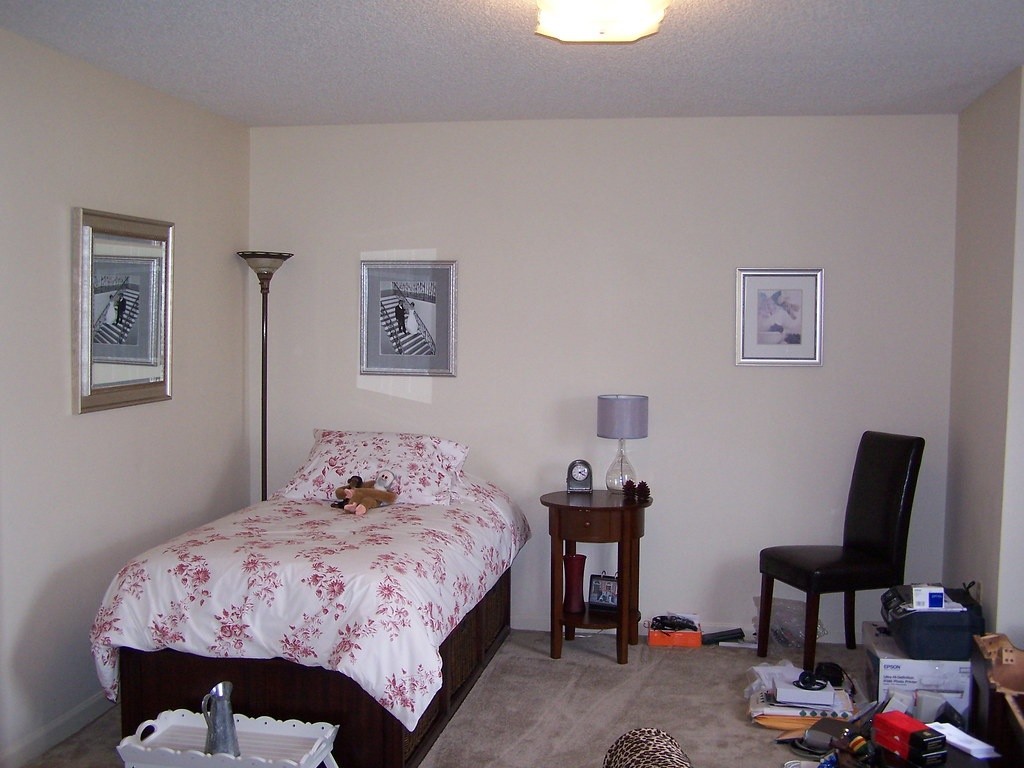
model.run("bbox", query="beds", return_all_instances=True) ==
[88,471,532,768]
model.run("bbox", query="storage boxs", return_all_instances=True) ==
[861,621,971,731]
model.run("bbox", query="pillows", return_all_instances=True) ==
[270,429,469,506]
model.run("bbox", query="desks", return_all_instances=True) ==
[116,709,341,768]
[540,489,653,665]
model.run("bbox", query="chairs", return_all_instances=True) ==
[757,429,925,673]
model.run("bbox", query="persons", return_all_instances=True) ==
[104,293,126,323]
[395,300,419,335]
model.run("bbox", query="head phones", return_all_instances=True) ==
[794,671,826,691]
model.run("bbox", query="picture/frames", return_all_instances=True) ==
[735,268,824,367]
[71,206,175,415]
[360,259,458,377]
[589,574,617,609]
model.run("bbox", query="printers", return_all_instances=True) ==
[881,583,986,661]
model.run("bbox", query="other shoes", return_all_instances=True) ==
[404,331,407,334]
[399,330,402,333]
[115,322,119,325]
[120,321,122,324]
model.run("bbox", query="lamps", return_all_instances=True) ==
[597,395,648,494]
[237,251,295,502]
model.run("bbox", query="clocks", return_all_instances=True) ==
[567,460,594,495]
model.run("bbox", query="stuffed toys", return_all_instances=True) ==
[330,470,397,516]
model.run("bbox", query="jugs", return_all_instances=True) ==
[202,681,241,757]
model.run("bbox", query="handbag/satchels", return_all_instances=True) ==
[802,718,861,751]
